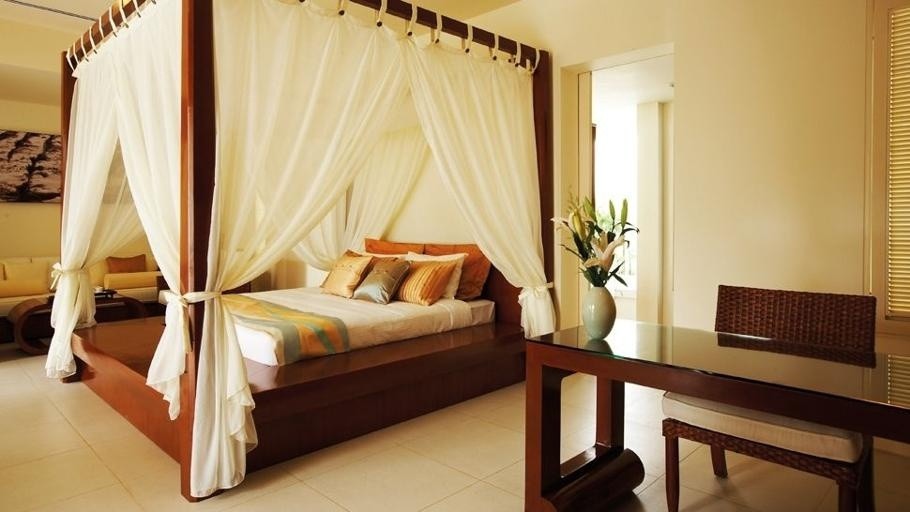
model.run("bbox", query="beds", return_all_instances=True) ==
[43,1,562,503]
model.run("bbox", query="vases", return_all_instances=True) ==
[580,286,617,339]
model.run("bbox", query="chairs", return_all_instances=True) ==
[660,284,878,512]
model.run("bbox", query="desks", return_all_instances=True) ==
[522,321,909,511]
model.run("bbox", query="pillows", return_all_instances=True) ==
[404,251,469,300]
[4,263,49,279]
[104,271,162,290]
[0,280,49,297]
[320,252,372,298]
[395,260,457,306]
[423,242,492,302]
[352,260,410,304]
[358,248,408,260]
[363,235,423,254]
[104,253,145,274]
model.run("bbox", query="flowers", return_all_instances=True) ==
[550,191,640,286]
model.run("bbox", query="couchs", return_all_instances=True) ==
[1,256,161,344]
